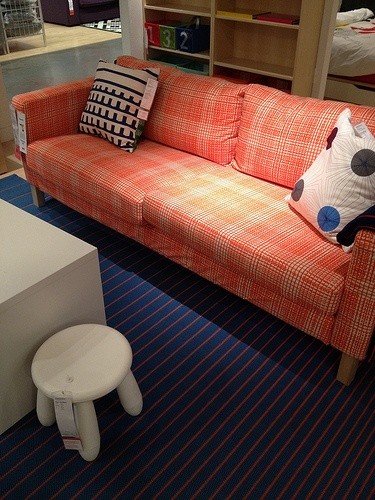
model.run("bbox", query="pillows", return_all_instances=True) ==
[283,107,375,252]
[79,57,159,152]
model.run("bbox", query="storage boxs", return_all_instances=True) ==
[145,20,175,46]
[159,23,191,49]
[176,25,210,53]
[147,52,291,95]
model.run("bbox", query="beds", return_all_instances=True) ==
[328,9,375,84]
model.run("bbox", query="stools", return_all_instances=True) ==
[31,324,143,462]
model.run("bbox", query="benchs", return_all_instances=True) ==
[11,54,375,386]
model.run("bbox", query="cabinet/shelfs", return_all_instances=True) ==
[0,0,47,54]
[143,0,326,98]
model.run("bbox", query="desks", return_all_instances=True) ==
[0,197,106,436]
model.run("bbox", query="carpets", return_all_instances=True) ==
[81,17,121,33]
[0,173,375,500]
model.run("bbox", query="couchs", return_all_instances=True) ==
[40,0,120,27]
